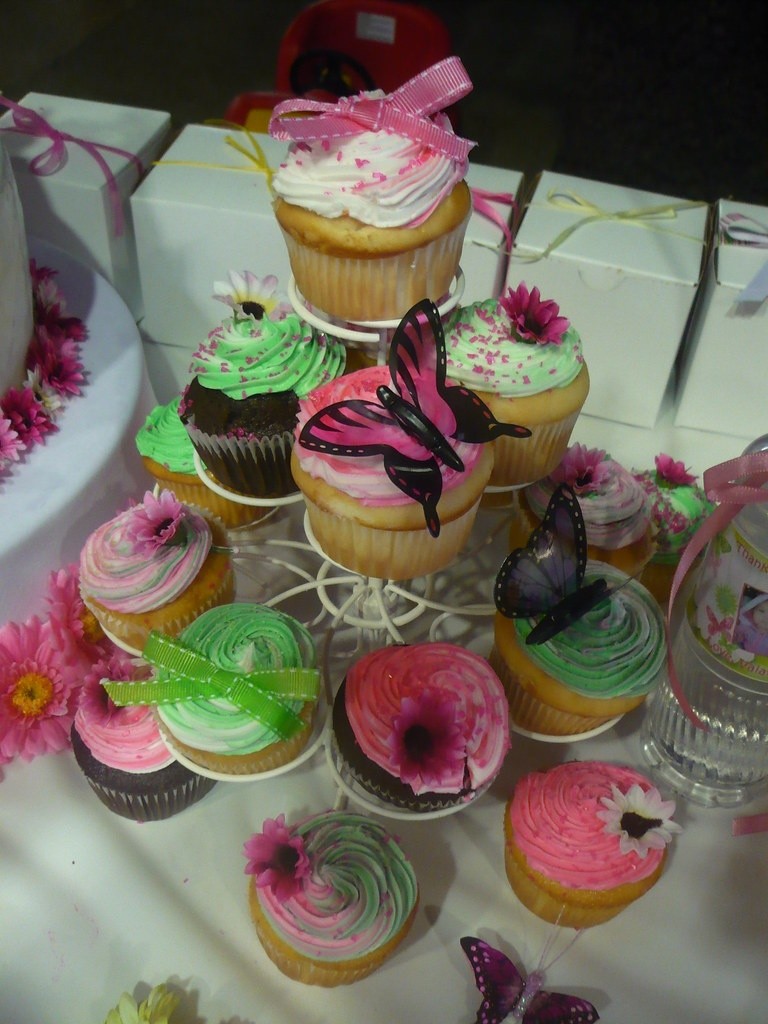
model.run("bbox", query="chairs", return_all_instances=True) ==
[227,0,451,125]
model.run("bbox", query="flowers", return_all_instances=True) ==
[498,281,572,348]
[210,269,294,324]
[654,452,699,489]
[242,812,314,904]
[125,489,192,562]
[595,781,684,861]
[549,441,624,500]
[0,258,90,468]
[0,563,148,784]
[105,983,180,1024]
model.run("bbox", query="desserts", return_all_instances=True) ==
[174,271,589,580]
[69,386,717,987]
[267,56,477,320]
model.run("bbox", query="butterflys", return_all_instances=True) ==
[299,298,532,538]
[493,481,646,647]
[459,901,601,1024]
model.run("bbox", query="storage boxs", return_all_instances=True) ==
[458,164,524,304]
[503,170,708,431]
[674,198,767,438]
[128,124,294,349]
[0,91,175,324]
